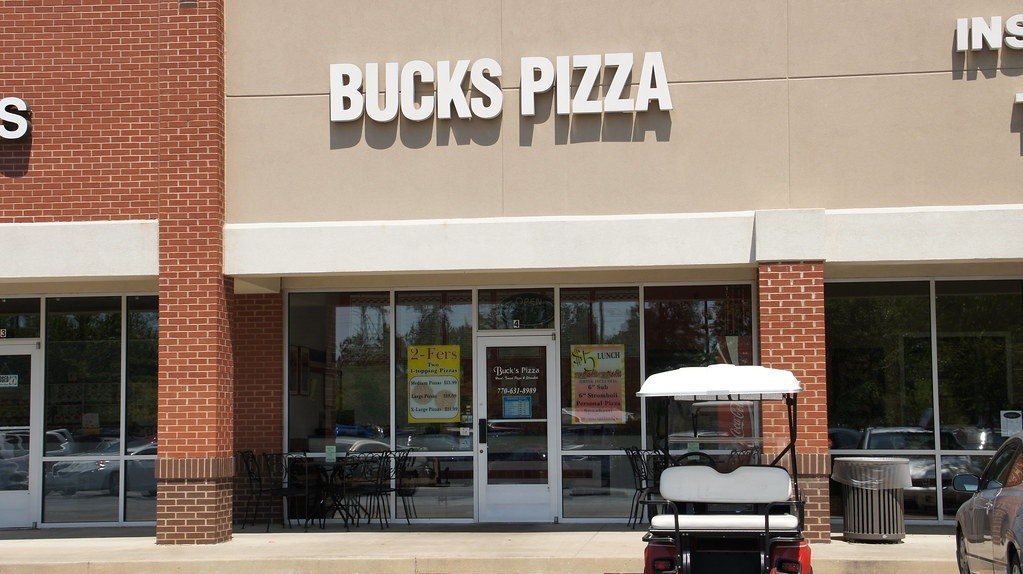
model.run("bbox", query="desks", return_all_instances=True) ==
[290,462,364,531]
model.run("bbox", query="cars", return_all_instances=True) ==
[0,425,158,501]
[830,424,1008,517]
[307,419,749,498]
[952,429,1023,574]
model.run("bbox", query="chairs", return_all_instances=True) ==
[619,445,763,529]
[236,448,418,531]
[876,439,895,449]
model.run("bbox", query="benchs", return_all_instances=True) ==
[650,466,799,530]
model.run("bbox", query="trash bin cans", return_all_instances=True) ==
[834,457,910,543]
